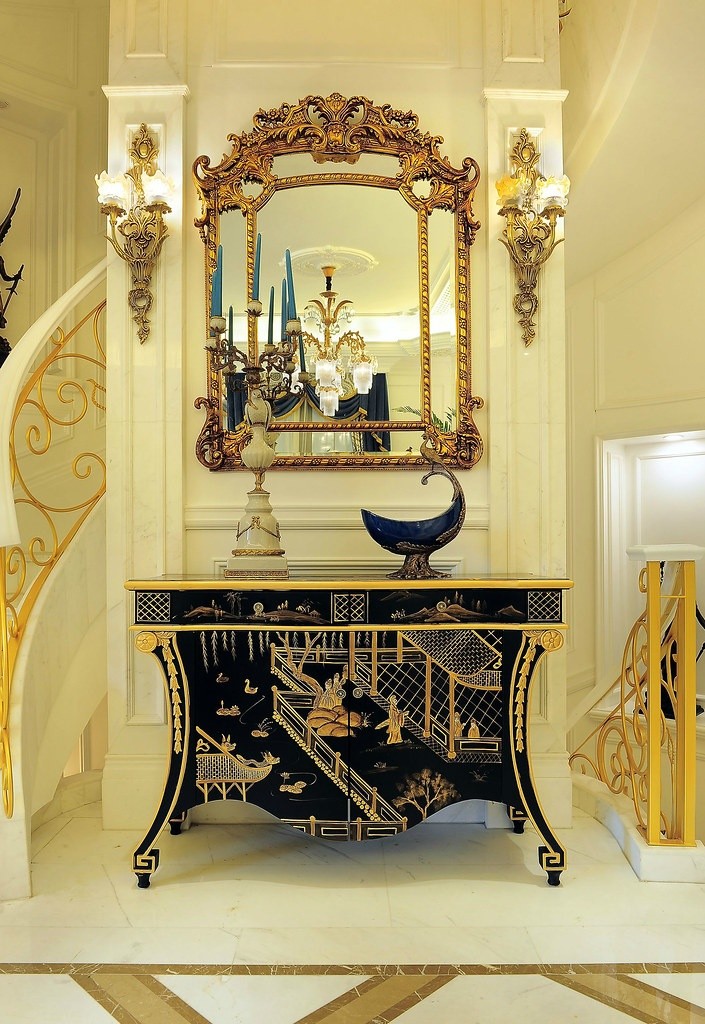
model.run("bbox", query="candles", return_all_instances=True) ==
[211,230,301,375]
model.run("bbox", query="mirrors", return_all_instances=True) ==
[193,90,485,472]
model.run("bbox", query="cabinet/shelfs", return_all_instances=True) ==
[120,574,572,883]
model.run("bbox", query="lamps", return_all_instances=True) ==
[278,246,379,416]
[491,127,571,347]
[92,122,176,345]
